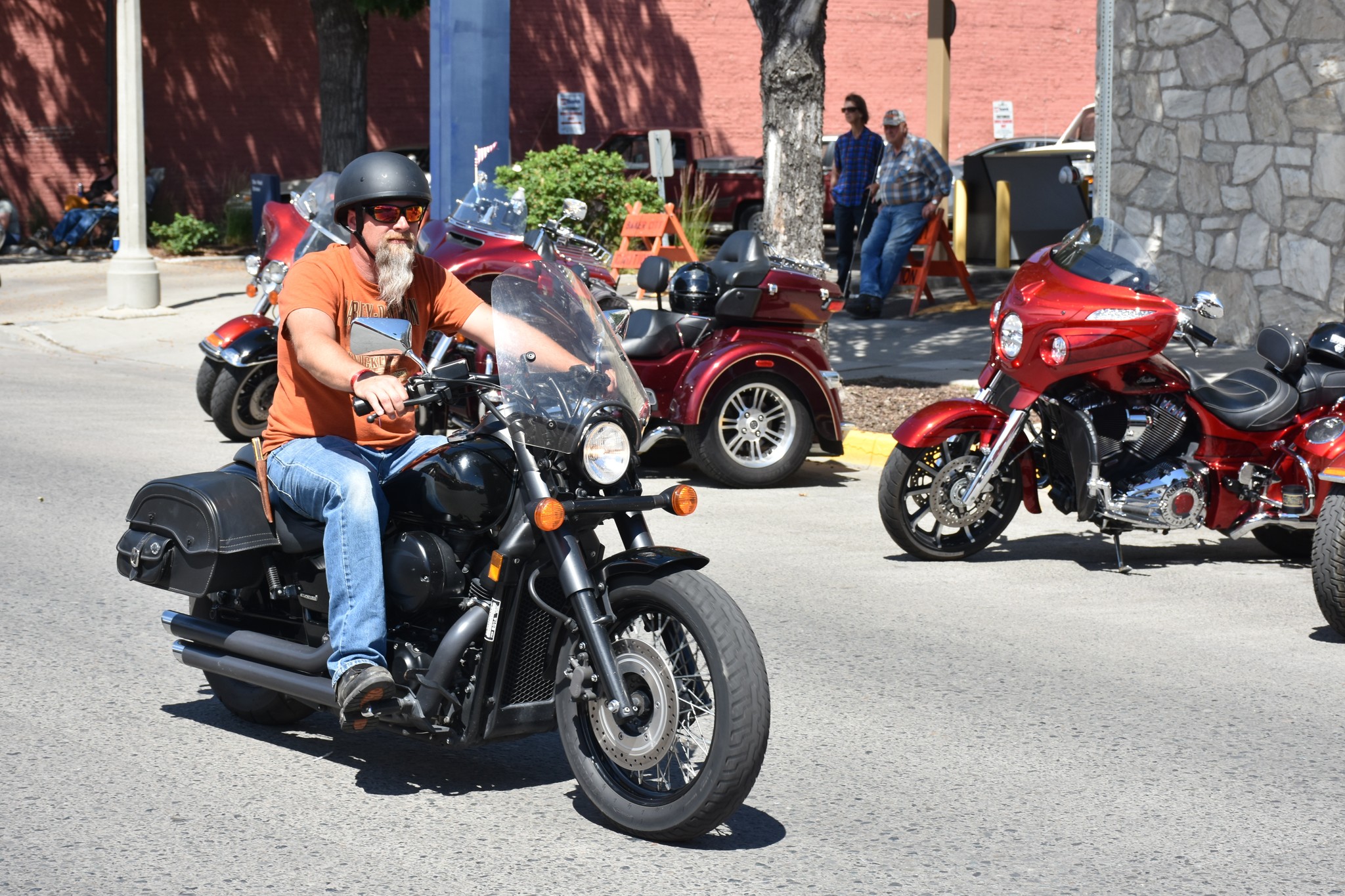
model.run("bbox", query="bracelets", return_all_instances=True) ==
[351,368,374,393]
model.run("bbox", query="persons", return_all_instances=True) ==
[0,185,21,254]
[829,94,885,298]
[76,150,118,244]
[844,109,953,319]
[258,151,621,730]
[36,150,156,256]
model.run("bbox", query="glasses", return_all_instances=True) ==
[362,203,428,223]
[841,107,857,113]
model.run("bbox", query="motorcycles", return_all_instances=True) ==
[196,169,623,442]
[414,181,847,490]
[875,215,1345,636]
[115,257,771,844]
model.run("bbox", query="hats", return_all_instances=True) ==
[882,110,906,127]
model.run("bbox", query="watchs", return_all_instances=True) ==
[931,199,939,206]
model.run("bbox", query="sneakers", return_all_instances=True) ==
[339,664,395,735]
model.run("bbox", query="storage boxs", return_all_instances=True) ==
[114,470,276,602]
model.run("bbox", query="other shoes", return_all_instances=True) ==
[845,296,881,319]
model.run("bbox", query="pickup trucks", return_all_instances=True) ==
[594,127,836,240]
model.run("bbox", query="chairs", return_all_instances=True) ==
[675,228,764,333]
[590,253,675,363]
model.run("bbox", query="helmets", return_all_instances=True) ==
[334,150,433,225]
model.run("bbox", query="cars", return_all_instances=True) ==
[821,102,1096,231]
